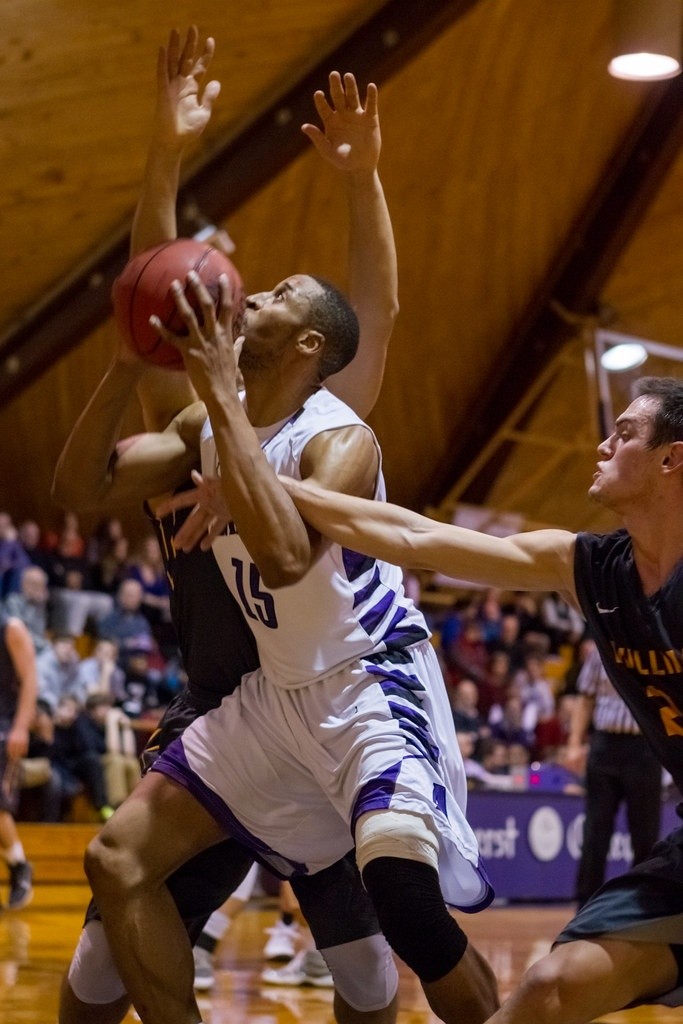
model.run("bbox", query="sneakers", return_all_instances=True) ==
[264,919,300,960]
[261,944,334,987]
[192,945,216,990]
[8,862,32,906]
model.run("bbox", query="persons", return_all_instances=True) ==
[156,375,683,1024]
[57,22,402,1024]
[50,270,500,1024]
[0,510,670,989]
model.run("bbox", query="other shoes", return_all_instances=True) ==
[99,804,115,821]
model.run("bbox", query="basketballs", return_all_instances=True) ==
[111,239,244,373]
